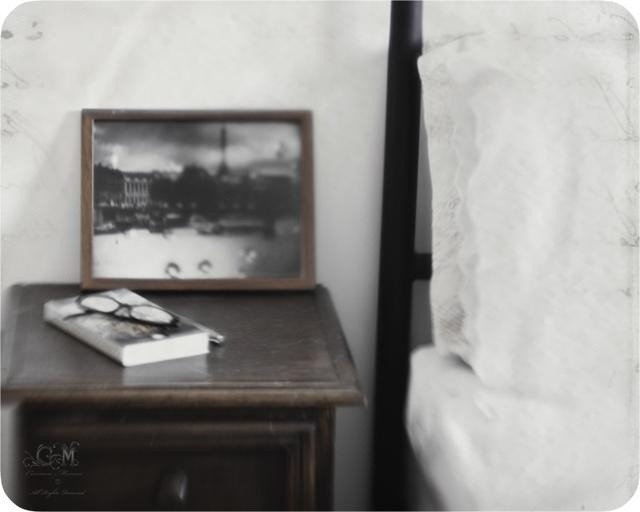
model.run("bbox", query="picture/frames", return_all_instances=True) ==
[80,107,316,292]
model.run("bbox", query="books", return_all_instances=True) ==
[43,286,210,367]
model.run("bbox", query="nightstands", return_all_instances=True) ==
[0,282,365,512]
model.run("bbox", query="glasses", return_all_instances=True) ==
[62,289,180,330]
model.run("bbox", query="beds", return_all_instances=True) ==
[370,0,640,511]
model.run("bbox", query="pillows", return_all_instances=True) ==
[419,31,640,398]
